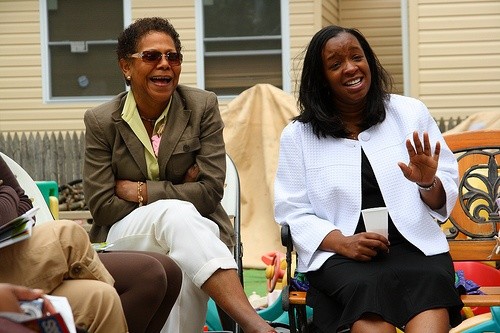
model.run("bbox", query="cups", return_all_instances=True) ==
[361,207,390,242]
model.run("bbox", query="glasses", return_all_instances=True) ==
[126,50,183,66]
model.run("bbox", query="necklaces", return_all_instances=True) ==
[140,116,156,126]
[348,132,360,140]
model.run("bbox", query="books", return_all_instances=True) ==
[0,156,183,333]
[0,295,76,333]
[0,204,44,247]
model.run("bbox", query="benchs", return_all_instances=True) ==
[279,131,500,333]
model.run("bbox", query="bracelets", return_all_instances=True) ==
[137,181,144,207]
[415,180,436,191]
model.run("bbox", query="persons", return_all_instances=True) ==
[274,25,462,333]
[84,16,279,333]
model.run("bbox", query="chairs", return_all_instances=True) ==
[0,153,246,333]
